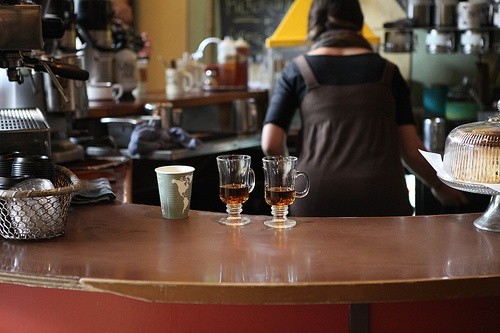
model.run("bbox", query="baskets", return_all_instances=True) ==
[0,164,81,240]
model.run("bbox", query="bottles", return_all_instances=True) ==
[407,0,500,29]
[215,34,251,88]
[424,117,445,151]
[231,97,259,137]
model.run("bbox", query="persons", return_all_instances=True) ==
[261,1,428,218]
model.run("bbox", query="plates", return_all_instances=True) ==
[0,151,55,180]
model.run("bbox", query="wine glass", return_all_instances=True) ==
[262,155,310,229]
[217,155,256,226]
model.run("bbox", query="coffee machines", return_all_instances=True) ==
[0,1,144,190]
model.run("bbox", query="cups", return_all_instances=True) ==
[156,165,195,218]
[164,60,205,96]
[86,81,123,101]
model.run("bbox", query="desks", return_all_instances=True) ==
[0,89,499,333]
